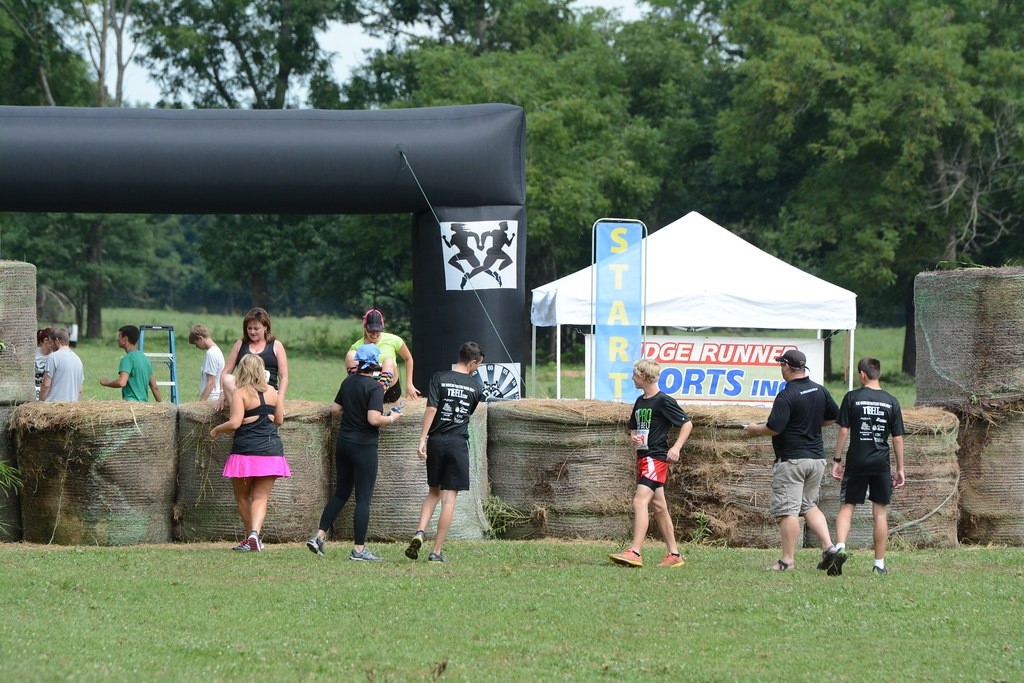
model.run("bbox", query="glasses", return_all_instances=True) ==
[780,361,788,366]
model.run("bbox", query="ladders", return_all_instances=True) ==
[138,325,179,405]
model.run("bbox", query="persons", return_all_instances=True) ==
[827,355,905,576]
[34,327,53,404]
[745,350,839,571]
[211,306,289,411]
[99,325,162,402]
[210,353,292,551]
[345,309,421,403]
[405,341,485,562]
[307,343,403,560]
[607,359,693,566]
[39,326,85,403]
[188,323,225,402]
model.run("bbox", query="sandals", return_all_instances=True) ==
[816,545,837,570]
[766,560,794,571]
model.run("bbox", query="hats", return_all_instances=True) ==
[775,350,807,368]
[363,310,384,333]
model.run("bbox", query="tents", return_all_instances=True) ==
[530,210,856,407]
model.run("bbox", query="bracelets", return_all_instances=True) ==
[833,456,842,463]
[389,417,393,424]
[220,389,223,392]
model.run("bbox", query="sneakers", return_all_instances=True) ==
[232,538,265,551]
[307,536,324,556]
[827,548,848,576]
[248,530,260,551]
[872,566,887,576]
[405,530,425,560]
[349,547,383,562]
[657,553,685,568]
[428,552,448,563]
[609,549,642,567]
